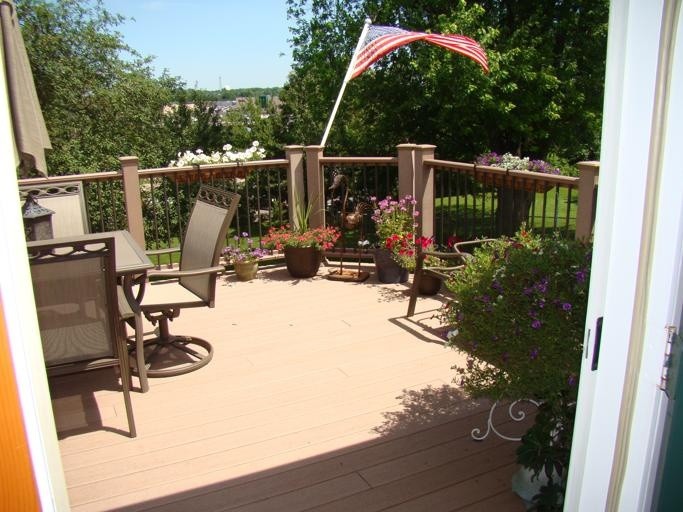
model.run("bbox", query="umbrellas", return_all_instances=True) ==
[0,0,53,180]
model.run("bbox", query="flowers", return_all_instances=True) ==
[472,150,561,176]
[224,222,342,263]
[368,191,467,272]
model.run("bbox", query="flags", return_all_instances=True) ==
[350,24,489,78]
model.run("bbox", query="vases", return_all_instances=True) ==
[168,164,256,183]
[418,275,442,296]
[235,260,259,280]
[369,246,409,284]
[283,245,322,278]
[167,140,267,171]
[473,163,556,193]
